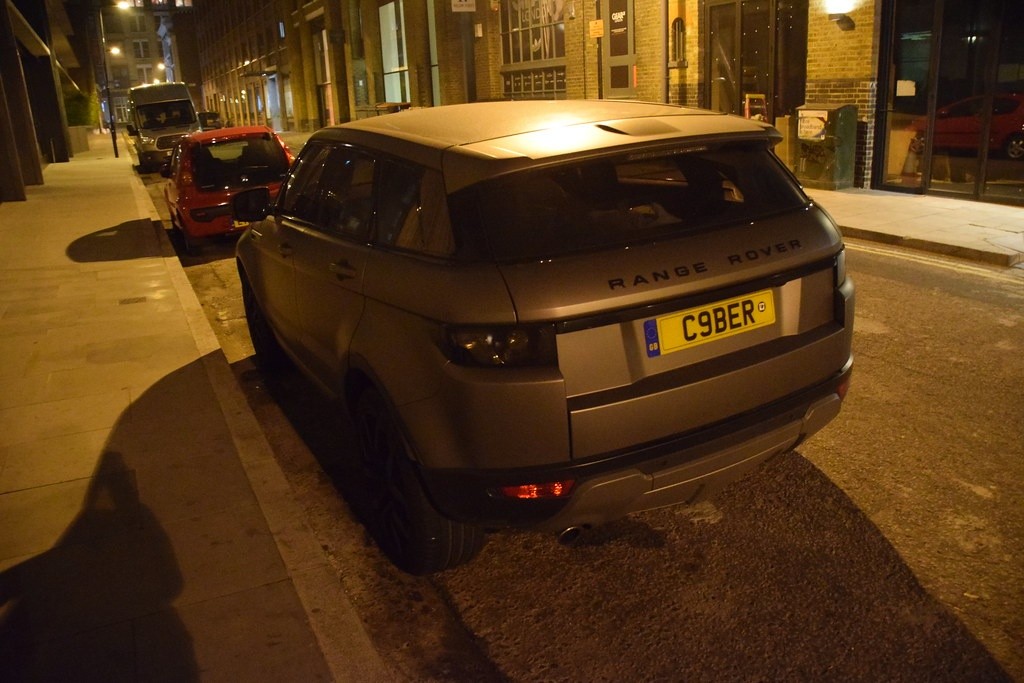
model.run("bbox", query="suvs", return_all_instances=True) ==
[226,97,859,577]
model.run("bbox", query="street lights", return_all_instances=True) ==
[97,0,130,158]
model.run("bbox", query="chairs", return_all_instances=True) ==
[144,113,161,128]
[163,111,180,126]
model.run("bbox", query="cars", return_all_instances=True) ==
[197,112,224,133]
[894,89,1024,163]
[160,125,299,257]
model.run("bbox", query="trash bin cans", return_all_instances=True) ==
[794,102,859,189]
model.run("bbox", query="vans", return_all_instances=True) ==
[125,81,204,174]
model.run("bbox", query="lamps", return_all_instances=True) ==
[191,146,222,177]
[241,145,266,165]
[827,12,854,32]
